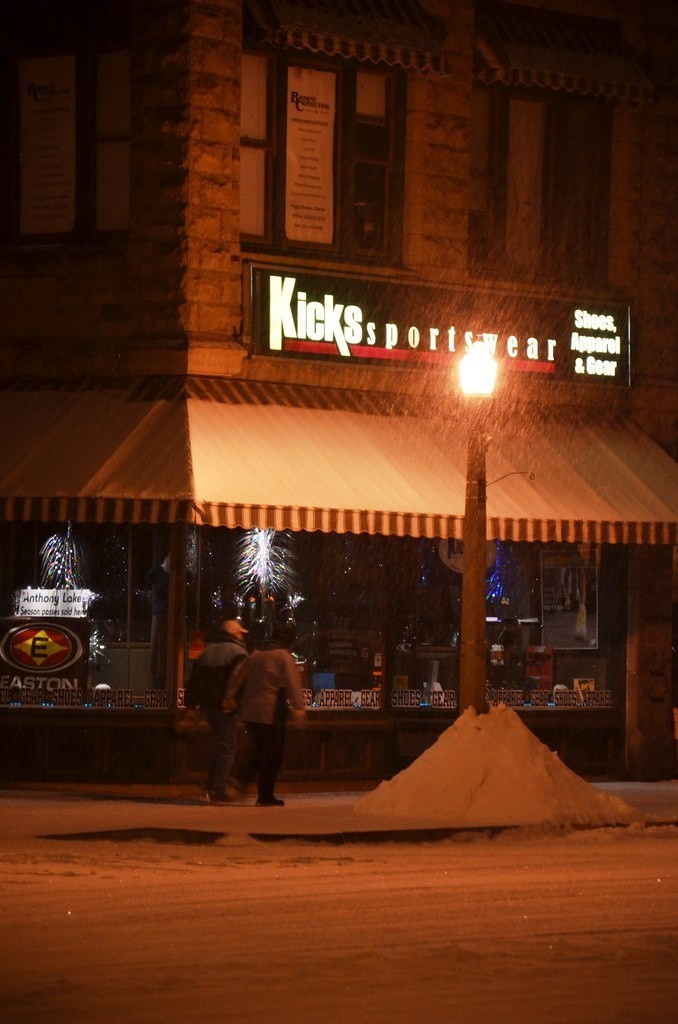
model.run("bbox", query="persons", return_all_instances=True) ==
[488,640,523,691]
[185,621,249,803]
[226,629,307,806]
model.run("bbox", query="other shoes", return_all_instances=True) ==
[209,786,235,802]
[255,795,284,805]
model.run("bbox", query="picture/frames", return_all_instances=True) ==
[533,543,605,656]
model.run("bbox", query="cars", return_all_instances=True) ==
[282,628,408,692]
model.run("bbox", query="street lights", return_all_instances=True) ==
[455,342,502,718]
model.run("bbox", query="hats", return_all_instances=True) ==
[222,620,249,635]
[273,623,302,643]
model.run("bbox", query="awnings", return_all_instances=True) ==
[1,376,678,544]
